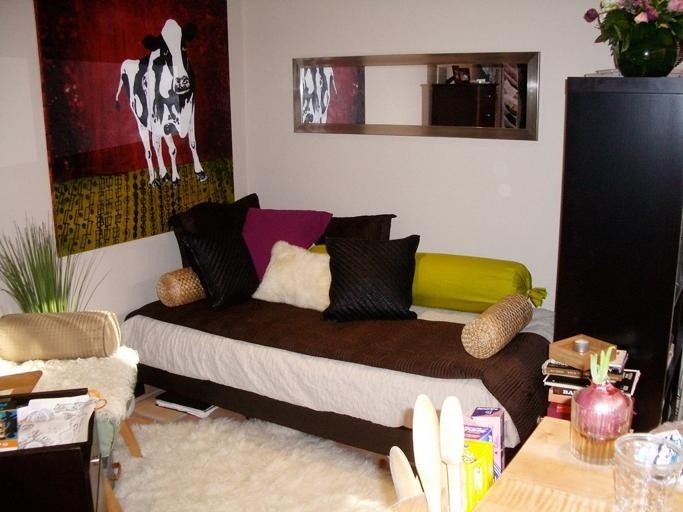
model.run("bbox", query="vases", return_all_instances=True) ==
[613,29,677,77]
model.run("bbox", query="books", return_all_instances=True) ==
[541,349,640,421]
[155,392,219,419]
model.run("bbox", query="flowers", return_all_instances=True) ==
[584,1,683,56]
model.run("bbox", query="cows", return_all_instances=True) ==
[300,65,338,124]
[115,17,207,188]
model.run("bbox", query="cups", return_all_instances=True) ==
[570,389,633,465]
[573,340,590,352]
[616,433,683,512]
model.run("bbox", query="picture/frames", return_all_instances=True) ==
[447,65,460,83]
[459,68,470,82]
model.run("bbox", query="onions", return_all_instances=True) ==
[575,345,631,437]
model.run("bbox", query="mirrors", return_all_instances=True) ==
[291,52,539,139]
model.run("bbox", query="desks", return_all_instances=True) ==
[464,416,681,512]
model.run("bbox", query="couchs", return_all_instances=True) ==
[122,191,550,473]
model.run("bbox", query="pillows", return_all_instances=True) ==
[460,292,533,359]
[175,192,265,310]
[0,311,120,362]
[305,244,546,315]
[242,206,332,283]
[255,241,332,312]
[316,212,395,248]
[323,235,420,320]
[158,266,208,307]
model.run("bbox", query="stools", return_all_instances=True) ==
[0,345,144,511]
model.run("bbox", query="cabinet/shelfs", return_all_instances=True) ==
[0,386,108,512]
[433,82,499,127]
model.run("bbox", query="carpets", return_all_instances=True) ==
[106,418,391,511]
